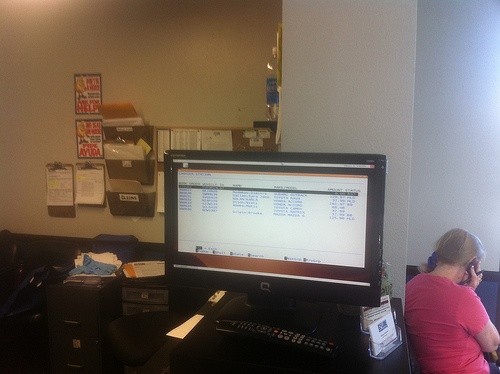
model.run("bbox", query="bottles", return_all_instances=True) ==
[265,47,279,120]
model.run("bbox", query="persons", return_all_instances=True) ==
[404,229,500,374]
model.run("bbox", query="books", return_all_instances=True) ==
[96,103,145,127]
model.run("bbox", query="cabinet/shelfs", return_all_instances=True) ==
[50,277,172,374]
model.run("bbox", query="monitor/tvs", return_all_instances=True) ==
[163,149,388,306]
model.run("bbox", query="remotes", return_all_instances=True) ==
[215,318,337,358]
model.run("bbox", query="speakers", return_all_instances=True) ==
[43,282,117,374]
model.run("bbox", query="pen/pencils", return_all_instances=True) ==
[458,269,486,285]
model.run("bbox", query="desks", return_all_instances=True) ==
[132,290,411,374]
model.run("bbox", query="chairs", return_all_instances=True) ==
[106,312,155,365]
